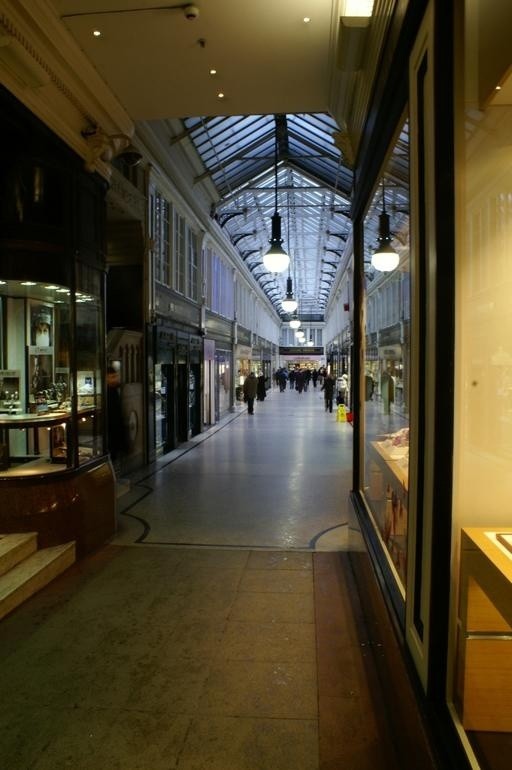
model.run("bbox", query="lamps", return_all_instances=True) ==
[263,116,314,347]
[371,175,400,272]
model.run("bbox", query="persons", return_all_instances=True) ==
[336,373,350,405]
[273,364,329,394]
[243,371,259,415]
[257,371,269,401]
[320,375,335,413]
